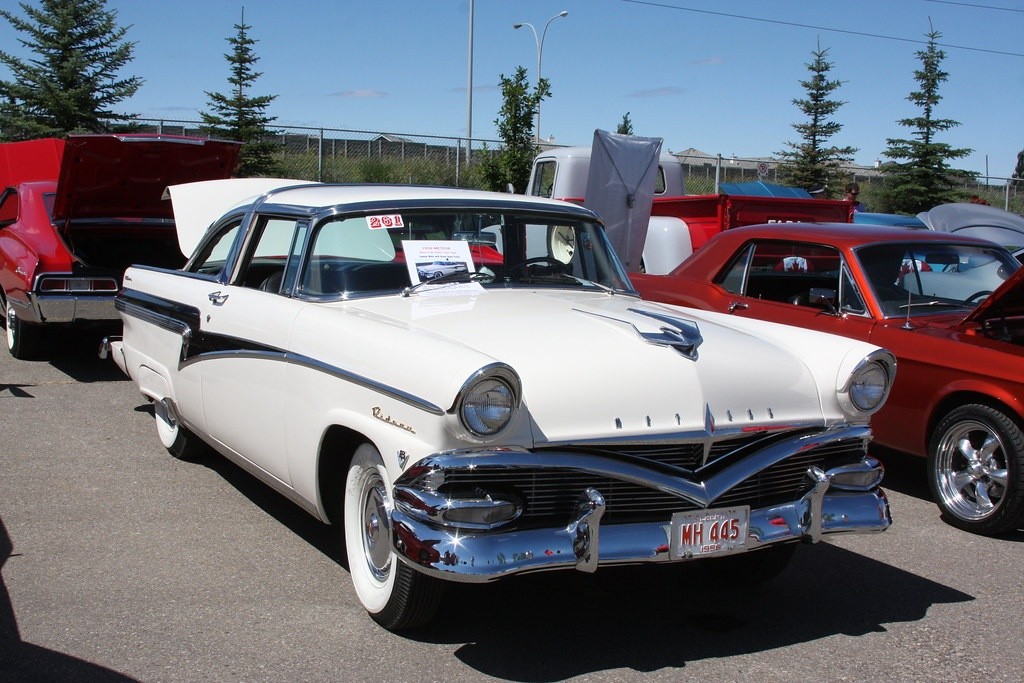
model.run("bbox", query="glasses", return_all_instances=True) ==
[850,192,860,195]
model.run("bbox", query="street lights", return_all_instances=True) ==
[513,10,569,143]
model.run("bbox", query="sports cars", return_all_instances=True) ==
[610,221,1024,539]
[99,175,899,636]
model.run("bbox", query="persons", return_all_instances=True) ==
[841,183,864,213]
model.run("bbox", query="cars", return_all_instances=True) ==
[0,133,247,362]
[467,144,1024,343]
